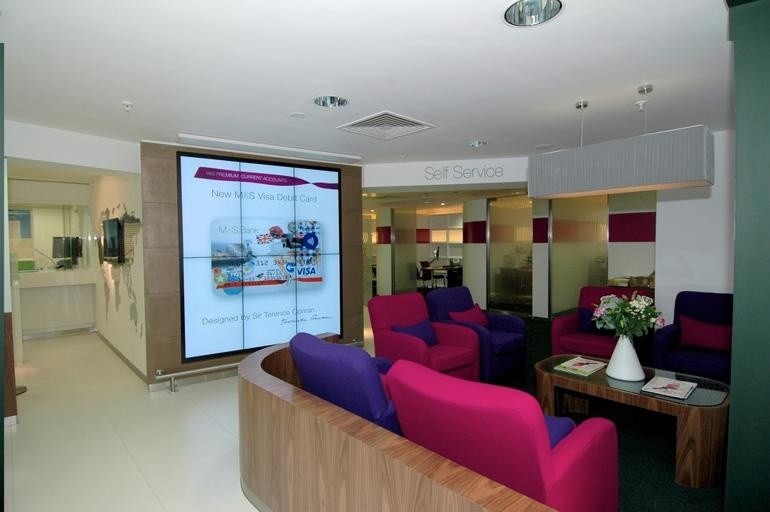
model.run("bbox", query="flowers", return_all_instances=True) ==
[592,291,664,343]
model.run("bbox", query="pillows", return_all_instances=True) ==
[392,321,438,347]
[544,416,576,447]
[449,303,488,327]
[578,309,616,335]
[679,315,730,349]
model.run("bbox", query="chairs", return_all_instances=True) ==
[551,286,649,358]
[644,291,733,382]
[420,260,445,288]
[389,360,618,511]
[292,332,400,436]
[426,286,529,385]
[369,292,477,382]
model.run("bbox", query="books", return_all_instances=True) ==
[642,375,691,400]
[670,379,699,399]
[560,356,609,373]
[553,355,606,377]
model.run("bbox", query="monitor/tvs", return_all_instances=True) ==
[103,218,125,263]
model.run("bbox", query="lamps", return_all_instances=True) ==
[417,246,439,280]
[527,84,716,199]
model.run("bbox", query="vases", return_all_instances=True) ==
[606,334,644,381]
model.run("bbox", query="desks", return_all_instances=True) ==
[424,266,461,288]
